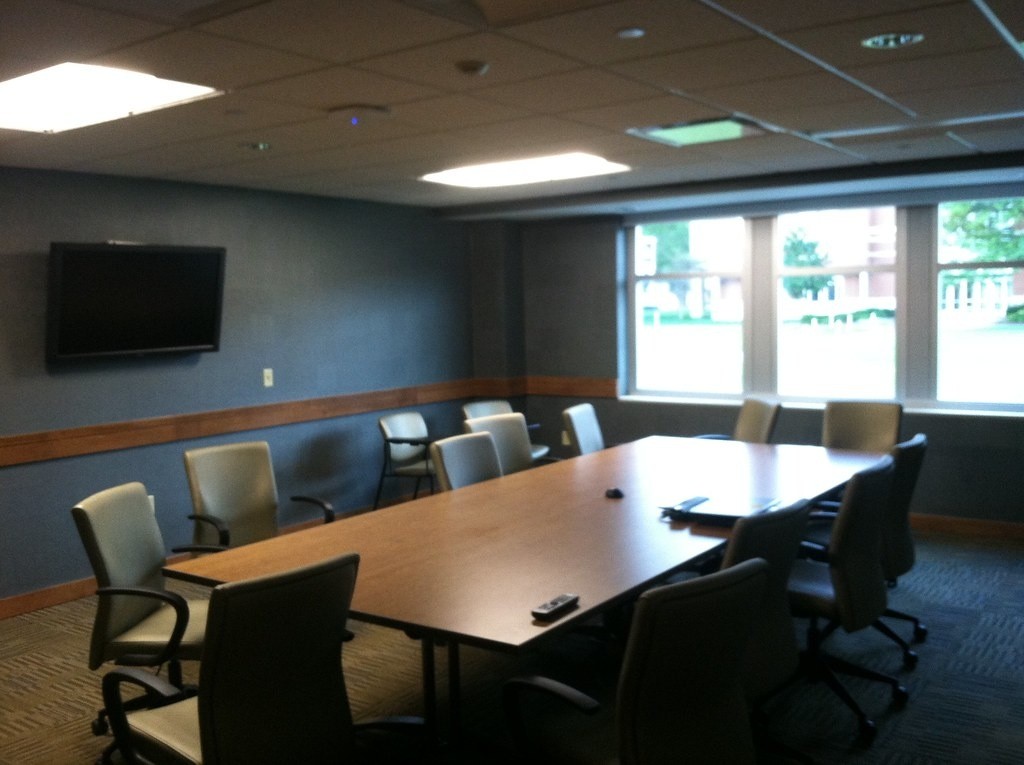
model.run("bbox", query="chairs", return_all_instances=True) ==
[171,438,356,642]
[372,410,436,510]
[102,551,361,765]
[431,398,926,765]
[70,482,212,735]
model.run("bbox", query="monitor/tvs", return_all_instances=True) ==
[44,243,226,360]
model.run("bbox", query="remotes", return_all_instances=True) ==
[531,592,579,621]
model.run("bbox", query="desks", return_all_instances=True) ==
[160,437,892,765]
